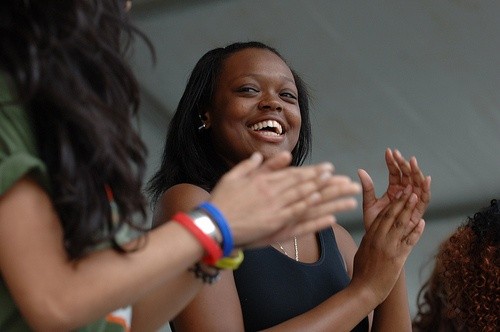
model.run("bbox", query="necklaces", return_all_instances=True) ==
[277,237,298,262]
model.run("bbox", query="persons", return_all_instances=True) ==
[411,199,500,332]
[0,0,362,332]
[145,42,432,332]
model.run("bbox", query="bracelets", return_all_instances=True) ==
[188,263,220,285]
[171,201,244,271]
